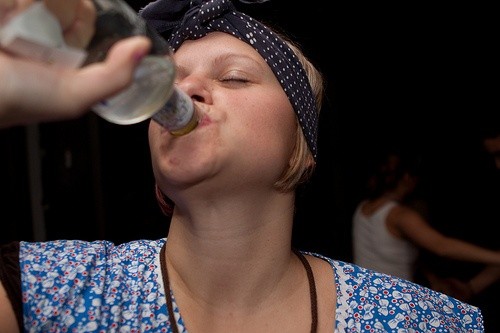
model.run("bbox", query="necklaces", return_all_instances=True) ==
[161,240,320,332]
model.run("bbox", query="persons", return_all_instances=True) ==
[1,0,485,333]
[354,146,500,281]
[458,263,500,302]
[448,122,500,333]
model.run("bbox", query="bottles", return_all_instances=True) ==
[0,0,200,135]
